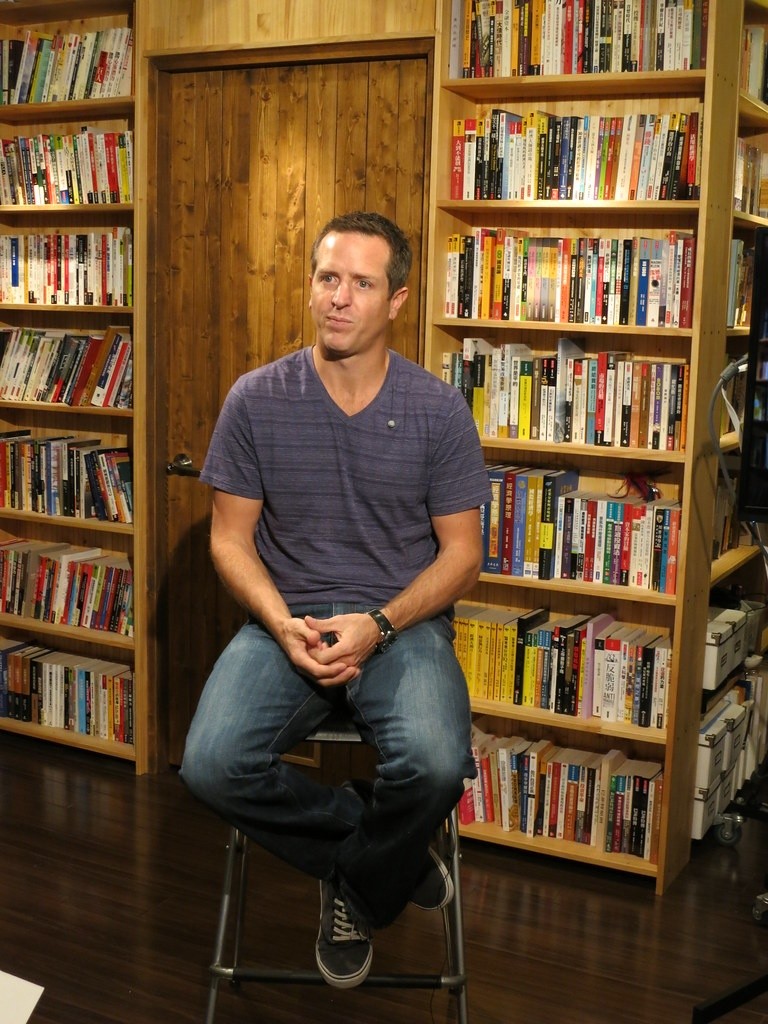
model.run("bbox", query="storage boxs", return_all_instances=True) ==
[690,606,745,839]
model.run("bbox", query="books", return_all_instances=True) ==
[435,0,768,866]
[0,25,135,748]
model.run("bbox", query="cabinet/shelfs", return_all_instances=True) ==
[423,0,745,896]
[0,0,147,776]
[690,0,768,840]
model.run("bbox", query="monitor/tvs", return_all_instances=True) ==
[732,227,768,523]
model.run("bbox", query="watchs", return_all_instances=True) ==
[370,608,398,654]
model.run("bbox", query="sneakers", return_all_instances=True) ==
[314,873,373,988]
[404,843,453,911]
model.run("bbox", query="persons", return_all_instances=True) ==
[180,211,492,988]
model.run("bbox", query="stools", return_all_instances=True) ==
[206,696,467,1024]
